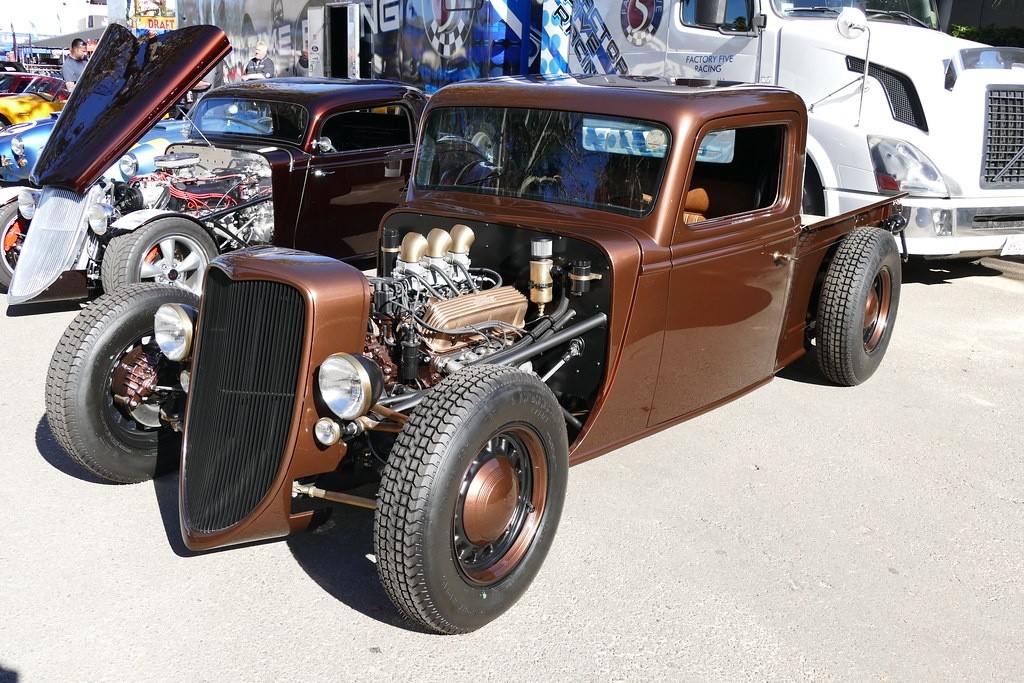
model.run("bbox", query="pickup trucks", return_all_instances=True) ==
[45,62,912,636]
[1,0,1023,314]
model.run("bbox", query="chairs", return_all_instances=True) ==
[627,179,709,226]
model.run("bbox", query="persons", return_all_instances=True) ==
[64,39,90,93]
[240,40,274,82]
